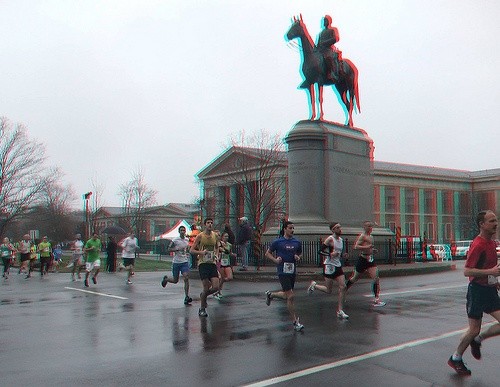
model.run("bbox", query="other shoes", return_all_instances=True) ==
[118,262,122,271]
[161,276,168,287]
[72,273,82,281]
[85,280,90,287]
[184,296,192,304]
[127,280,133,284]
[92,277,97,284]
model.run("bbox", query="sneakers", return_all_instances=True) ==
[306,281,316,295]
[369,299,386,307]
[337,311,349,319]
[447,355,472,376]
[292,322,304,329]
[265,290,271,306]
[199,307,208,317]
[470,338,482,360]
[214,293,223,300]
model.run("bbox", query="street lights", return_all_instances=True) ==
[84,191,92,240]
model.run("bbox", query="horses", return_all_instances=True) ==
[286,13,362,127]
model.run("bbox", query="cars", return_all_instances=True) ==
[415,245,446,261]
[443,245,451,260]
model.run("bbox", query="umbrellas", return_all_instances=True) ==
[101,226,126,235]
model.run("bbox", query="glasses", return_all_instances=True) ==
[207,222,213,224]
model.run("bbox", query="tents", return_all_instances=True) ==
[153,219,201,240]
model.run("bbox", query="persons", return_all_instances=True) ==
[298,15,343,90]
[307,223,349,320]
[265,221,304,331]
[0,217,252,317]
[448,210,500,375]
[345,221,386,307]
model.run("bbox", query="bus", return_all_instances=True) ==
[450,240,474,258]
